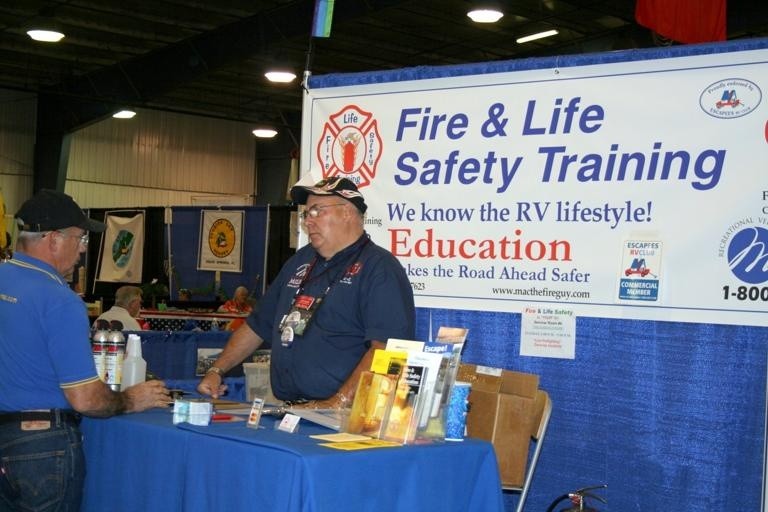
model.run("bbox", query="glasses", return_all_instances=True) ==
[68,235,90,245]
[300,204,345,220]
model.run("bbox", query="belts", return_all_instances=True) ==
[1,412,81,422]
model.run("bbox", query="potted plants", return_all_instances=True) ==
[179,288,192,301]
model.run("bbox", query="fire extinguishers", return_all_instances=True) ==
[546,484,608,512]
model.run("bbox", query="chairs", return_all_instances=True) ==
[515,390,553,512]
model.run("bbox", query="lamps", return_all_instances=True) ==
[111,106,137,120]
[467,0,505,23]
[251,123,278,139]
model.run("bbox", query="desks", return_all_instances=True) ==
[77,375,503,512]
[115,330,248,380]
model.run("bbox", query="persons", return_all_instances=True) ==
[92,286,144,333]
[0,190,176,511]
[282,328,291,346]
[196,177,417,415]
[176,287,192,303]
[225,286,253,332]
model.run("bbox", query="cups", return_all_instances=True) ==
[157,303,167,311]
[444,381,472,443]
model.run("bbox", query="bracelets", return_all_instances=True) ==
[336,393,353,407]
[206,367,223,377]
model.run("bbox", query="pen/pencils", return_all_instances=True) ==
[213,415,231,420]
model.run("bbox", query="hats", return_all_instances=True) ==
[291,178,367,214]
[14,189,106,233]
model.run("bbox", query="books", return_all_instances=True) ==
[283,405,351,431]
[348,325,472,444]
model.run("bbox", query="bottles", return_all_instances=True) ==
[120,336,148,393]
[91,318,109,385]
[125,334,137,354]
[106,320,125,393]
[211,318,219,331]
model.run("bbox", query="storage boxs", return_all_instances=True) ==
[453,363,540,487]
